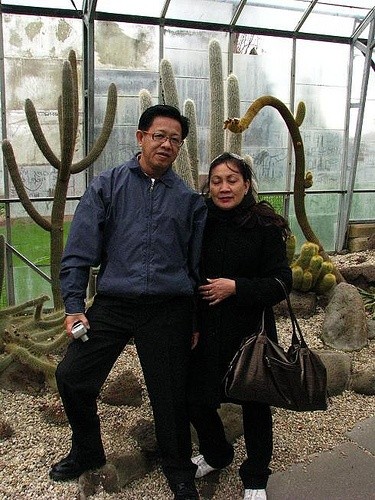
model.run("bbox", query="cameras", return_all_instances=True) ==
[72,321,89,342]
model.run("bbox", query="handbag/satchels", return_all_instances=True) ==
[221,277,328,412]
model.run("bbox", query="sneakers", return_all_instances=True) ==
[244,489,267,500]
[190,455,219,478]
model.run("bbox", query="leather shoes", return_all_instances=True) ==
[49,454,106,480]
[168,477,200,500]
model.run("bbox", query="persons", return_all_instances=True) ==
[49,105,207,500]
[190,151,292,500]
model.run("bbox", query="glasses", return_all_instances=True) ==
[142,130,184,147]
